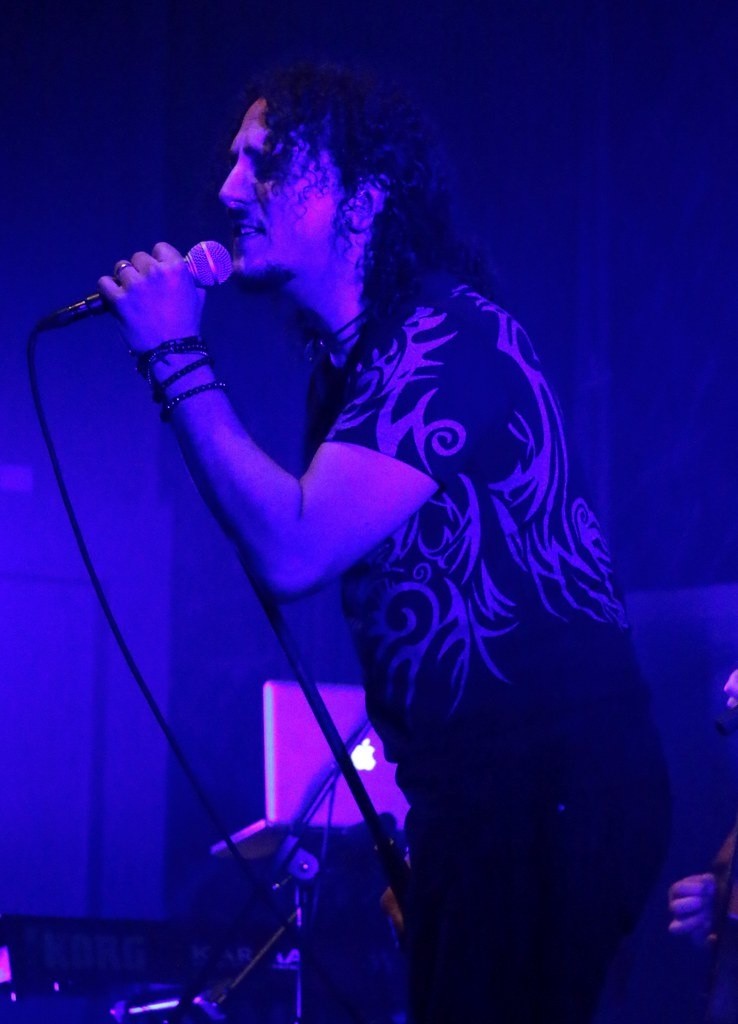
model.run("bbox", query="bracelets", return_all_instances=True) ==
[129,335,231,423]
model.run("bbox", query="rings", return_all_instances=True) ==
[116,263,132,281]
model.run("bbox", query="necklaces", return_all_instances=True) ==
[318,309,367,356]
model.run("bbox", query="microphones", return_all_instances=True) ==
[55,241,232,327]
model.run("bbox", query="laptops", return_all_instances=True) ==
[210,681,410,858]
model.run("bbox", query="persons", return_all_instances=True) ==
[670,672,738,1023]
[99,69,671,1023]
